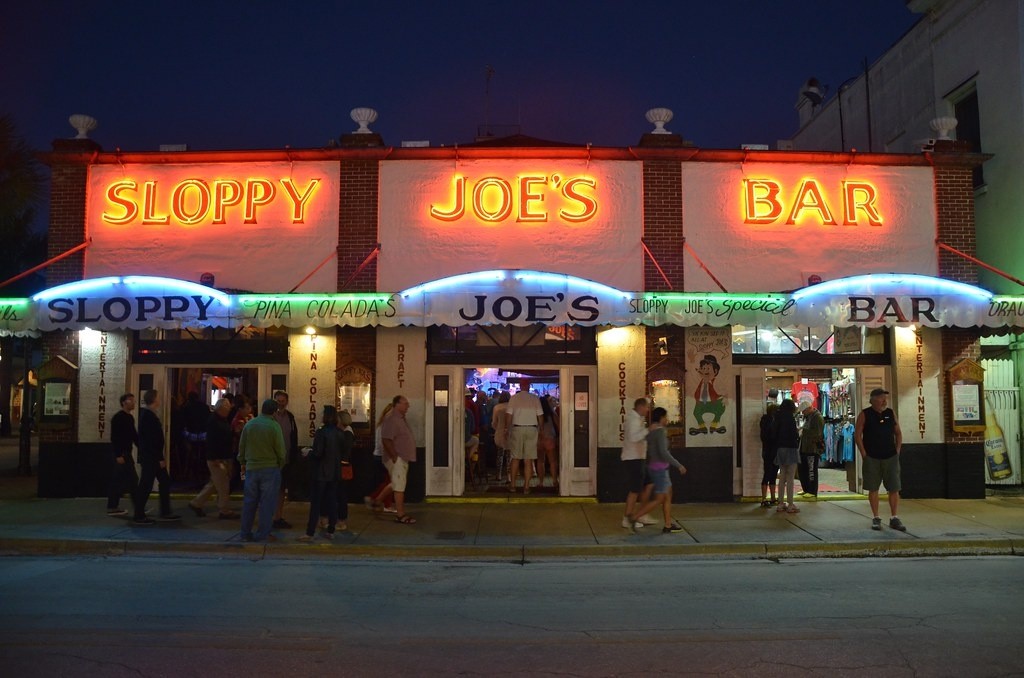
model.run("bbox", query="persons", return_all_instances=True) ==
[461,376,560,493]
[237,400,289,541]
[129,390,180,525]
[295,406,355,541]
[854,388,908,532]
[107,392,151,514]
[620,399,687,534]
[366,395,417,523]
[171,386,257,518]
[268,391,298,524]
[759,398,800,513]
[797,402,824,498]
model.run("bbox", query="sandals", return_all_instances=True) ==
[394,512,417,524]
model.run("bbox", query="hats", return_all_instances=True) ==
[870,389,889,396]
[800,402,811,412]
[465,387,472,396]
[477,391,487,399]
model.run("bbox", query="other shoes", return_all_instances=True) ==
[218,510,243,519]
[761,499,800,513]
[796,490,804,496]
[296,517,349,542]
[158,513,182,521]
[133,515,155,525]
[189,501,207,517]
[495,472,558,495]
[802,493,816,497]
[107,509,128,516]
[257,533,277,544]
[241,533,258,542]
[273,517,292,528]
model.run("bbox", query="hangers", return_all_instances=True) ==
[801,375,853,428]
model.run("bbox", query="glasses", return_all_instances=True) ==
[466,396,471,398]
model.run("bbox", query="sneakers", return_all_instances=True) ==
[889,518,906,531]
[872,517,882,530]
[621,513,683,533]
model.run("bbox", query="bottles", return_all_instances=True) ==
[983,393,1012,480]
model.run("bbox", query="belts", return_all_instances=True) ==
[513,425,537,427]
